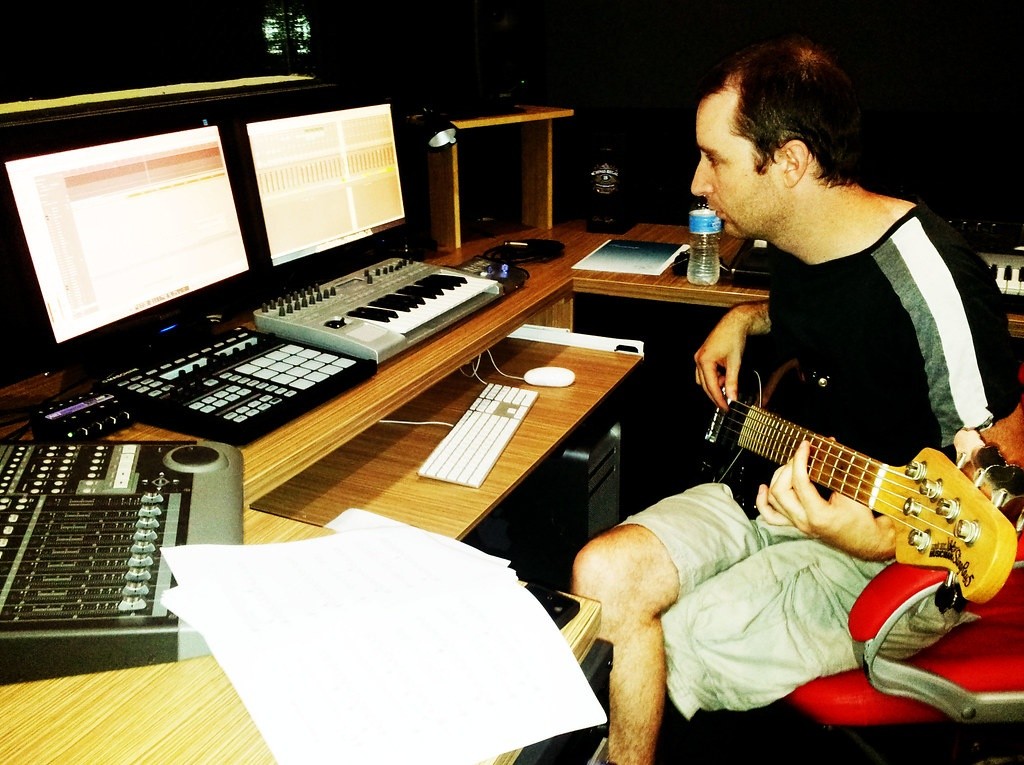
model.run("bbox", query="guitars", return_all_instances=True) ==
[699,367,1024,617]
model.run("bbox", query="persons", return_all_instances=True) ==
[572,37,1024,765]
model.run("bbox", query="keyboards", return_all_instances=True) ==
[416,383,539,489]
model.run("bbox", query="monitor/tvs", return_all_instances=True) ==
[0,82,426,379]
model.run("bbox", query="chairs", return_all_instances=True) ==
[781,362,1024,765]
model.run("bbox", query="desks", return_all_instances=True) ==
[0,210,1023,765]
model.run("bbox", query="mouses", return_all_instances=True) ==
[524,367,577,388]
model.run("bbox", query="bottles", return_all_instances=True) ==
[687,194,722,285]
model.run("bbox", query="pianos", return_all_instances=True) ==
[731,208,1024,315]
